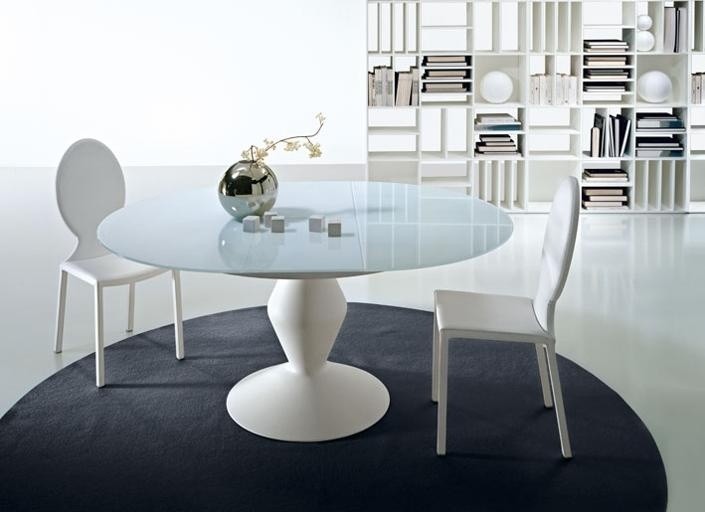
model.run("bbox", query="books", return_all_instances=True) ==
[532,70,579,109]
[582,36,633,210]
[692,72,705,105]
[368,62,417,107]
[635,112,685,157]
[664,5,689,53]
[420,54,474,94]
[474,110,523,155]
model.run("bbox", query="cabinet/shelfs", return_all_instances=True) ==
[363,0,705,216]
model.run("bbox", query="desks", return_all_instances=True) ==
[94,179,518,446]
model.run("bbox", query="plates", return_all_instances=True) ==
[638,15,652,30]
[637,72,672,101]
[480,70,514,103]
[637,30,655,51]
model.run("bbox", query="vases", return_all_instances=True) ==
[216,159,281,223]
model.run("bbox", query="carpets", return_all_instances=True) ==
[0,300,670,511]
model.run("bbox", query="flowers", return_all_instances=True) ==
[240,112,328,170]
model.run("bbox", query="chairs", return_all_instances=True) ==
[427,173,584,462]
[50,135,188,391]
